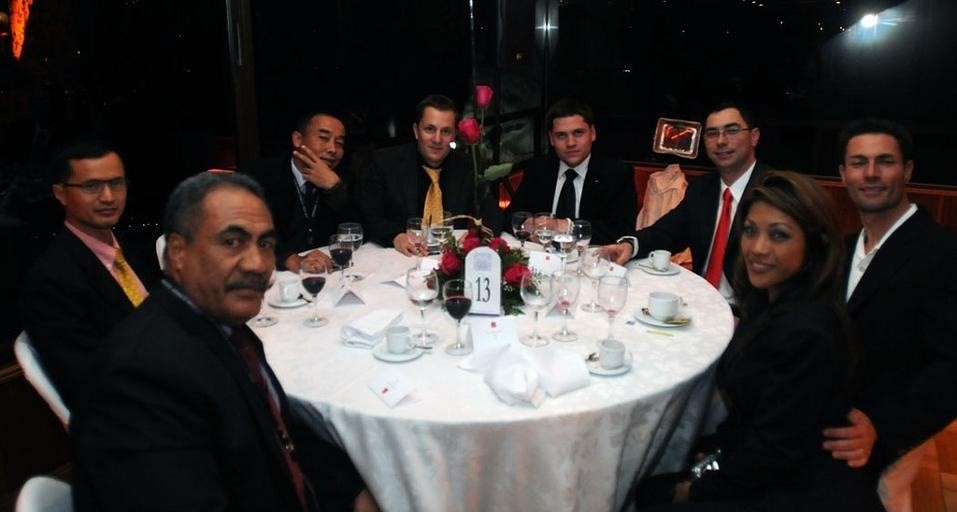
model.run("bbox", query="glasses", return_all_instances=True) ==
[63,178,129,194]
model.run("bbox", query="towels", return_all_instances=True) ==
[453,335,594,409]
[342,303,407,350]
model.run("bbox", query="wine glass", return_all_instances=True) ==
[442,279,474,358]
[330,234,354,288]
[578,245,612,312]
[551,269,581,341]
[553,226,579,265]
[512,211,533,248]
[533,215,558,253]
[405,217,429,273]
[337,222,362,265]
[569,220,592,262]
[597,275,627,338]
[251,265,279,328]
[300,256,329,329]
[520,270,550,347]
[406,269,439,347]
[428,212,454,262]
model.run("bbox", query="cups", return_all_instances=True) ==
[597,340,633,370]
[648,250,671,272]
[279,278,300,303]
[385,325,411,353]
[646,292,684,321]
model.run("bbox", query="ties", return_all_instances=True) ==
[230,327,309,511]
[114,248,144,308]
[304,181,317,218]
[556,169,578,221]
[420,165,443,229]
[705,187,733,290]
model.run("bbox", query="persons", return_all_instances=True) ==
[15,132,166,417]
[66,171,379,511]
[596,100,768,323]
[816,113,957,471]
[356,94,479,259]
[244,104,361,275]
[622,168,889,512]
[492,98,638,247]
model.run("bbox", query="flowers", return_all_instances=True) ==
[423,81,540,318]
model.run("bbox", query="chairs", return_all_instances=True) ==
[14,471,74,512]
[10,321,74,436]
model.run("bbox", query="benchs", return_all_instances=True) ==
[486,160,955,306]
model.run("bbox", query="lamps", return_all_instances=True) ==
[649,112,704,160]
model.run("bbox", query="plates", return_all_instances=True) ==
[632,307,692,327]
[372,344,424,361]
[269,299,309,308]
[640,258,680,274]
[585,359,631,375]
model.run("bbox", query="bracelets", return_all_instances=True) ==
[622,240,634,254]
